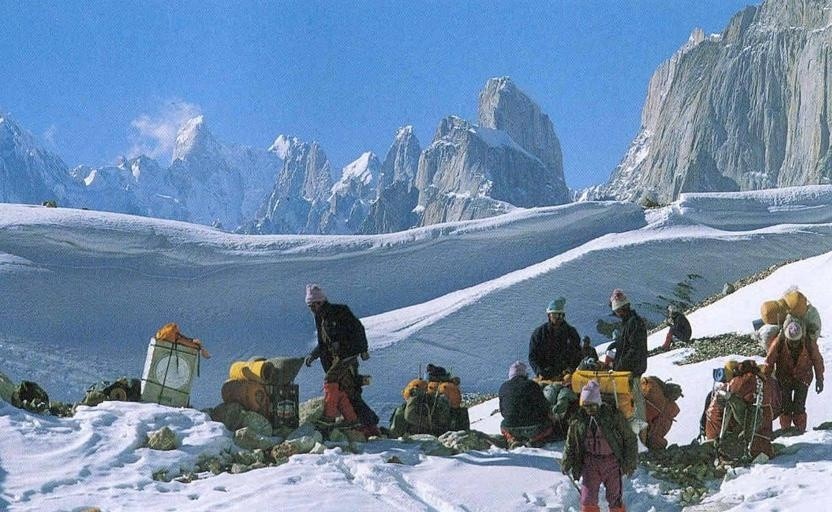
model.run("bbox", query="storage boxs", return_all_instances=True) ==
[256,382,301,432]
[131,335,200,410]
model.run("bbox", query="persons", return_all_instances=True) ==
[560,380,638,511]
[761,321,825,436]
[304,286,380,433]
[530,289,648,405]
[625,376,679,459]
[499,362,553,448]
[662,303,694,354]
[704,361,776,458]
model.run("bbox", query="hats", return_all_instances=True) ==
[305,285,325,306]
[509,360,527,379]
[546,296,566,315]
[785,322,803,341]
[580,381,602,408]
[611,289,631,312]
